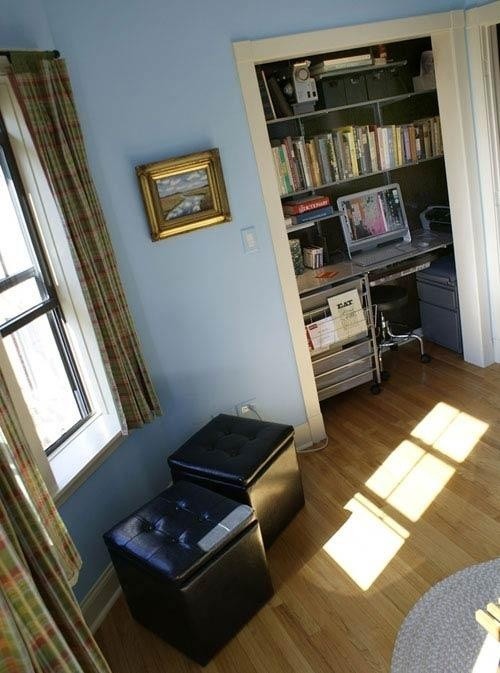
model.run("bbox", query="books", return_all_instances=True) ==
[309,55,373,77]
[279,198,334,228]
[257,70,293,121]
[271,115,444,196]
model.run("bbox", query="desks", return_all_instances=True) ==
[299,222,461,406]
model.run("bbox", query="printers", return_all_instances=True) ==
[419,205,452,232]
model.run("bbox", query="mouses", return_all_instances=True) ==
[417,240,430,248]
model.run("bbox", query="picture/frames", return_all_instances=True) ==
[135,146,232,243]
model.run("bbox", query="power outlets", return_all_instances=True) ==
[235,397,260,417]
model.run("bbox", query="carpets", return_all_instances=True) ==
[391,558,500,673]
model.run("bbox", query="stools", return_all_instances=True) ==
[167,413,305,551]
[370,285,431,381]
[102,480,275,667]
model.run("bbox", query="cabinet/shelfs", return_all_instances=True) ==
[298,274,383,405]
[415,256,462,353]
[257,50,445,234]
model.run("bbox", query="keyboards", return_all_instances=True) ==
[354,244,417,267]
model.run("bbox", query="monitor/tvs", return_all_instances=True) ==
[336,183,412,260]
